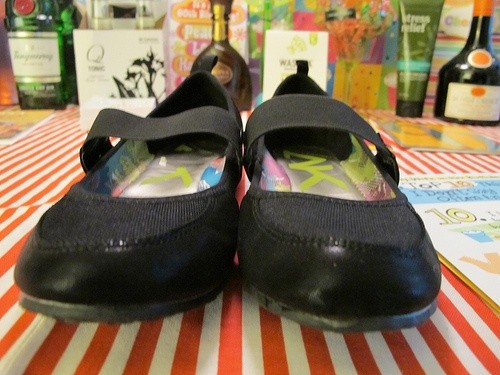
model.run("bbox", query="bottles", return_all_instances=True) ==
[3,0,82,112]
[433,0,500,126]
[189,0,254,114]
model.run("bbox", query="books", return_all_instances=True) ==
[398,172,500,318]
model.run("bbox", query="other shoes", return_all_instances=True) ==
[13,55,245,323]
[239,58,443,332]
